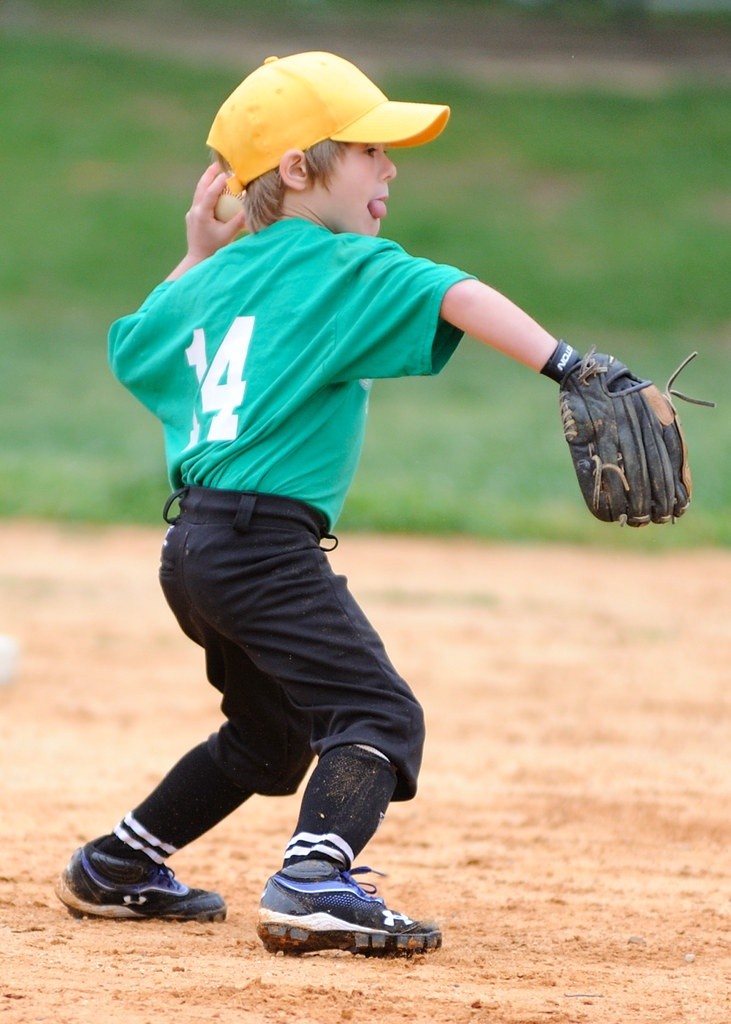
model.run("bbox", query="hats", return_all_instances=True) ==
[206,52,450,194]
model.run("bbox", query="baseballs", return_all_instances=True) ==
[214,171,248,229]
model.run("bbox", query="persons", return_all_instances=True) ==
[52,48,697,959]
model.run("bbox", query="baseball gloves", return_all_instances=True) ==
[560,344,716,530]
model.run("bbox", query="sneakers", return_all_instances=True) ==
[54,834,227,922]
[257,865,442,957]
